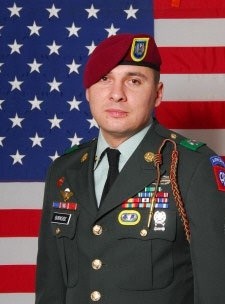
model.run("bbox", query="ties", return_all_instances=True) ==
[99,148,121,211]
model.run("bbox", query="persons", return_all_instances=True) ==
[35,34,225,304]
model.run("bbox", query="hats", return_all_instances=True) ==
[83,32,163,90]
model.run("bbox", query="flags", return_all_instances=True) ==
[0,0,225,304]
